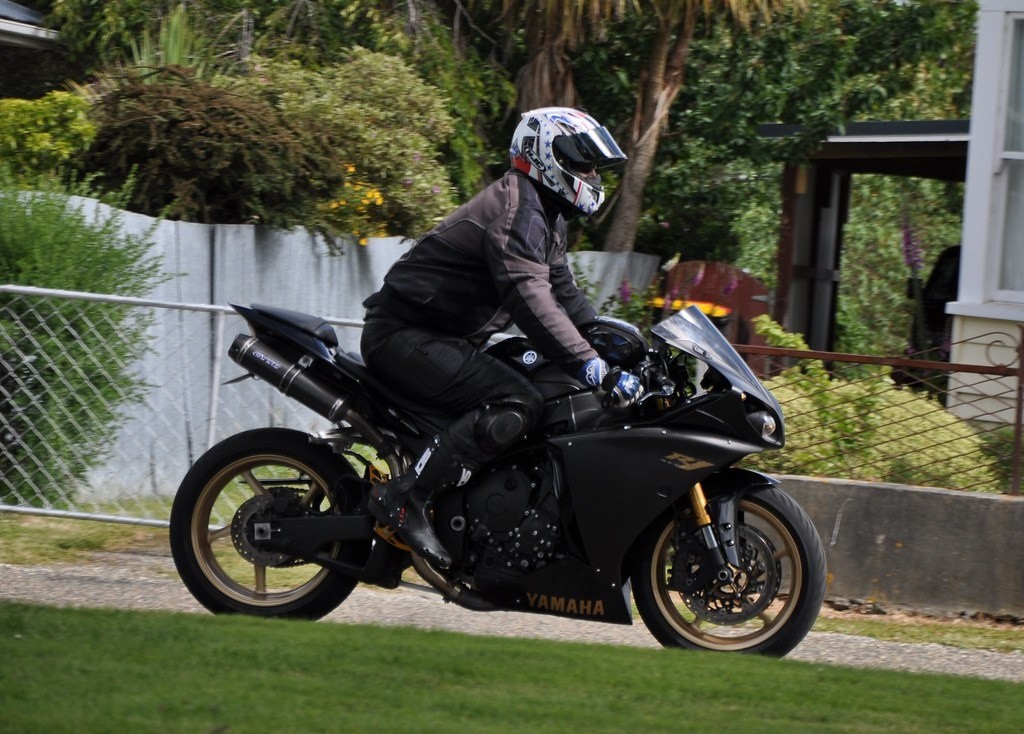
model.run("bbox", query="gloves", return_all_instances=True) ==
[580,357,643,407]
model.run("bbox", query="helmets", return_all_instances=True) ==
[511,106,628,216]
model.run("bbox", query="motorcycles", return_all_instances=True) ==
[169,304,827,663]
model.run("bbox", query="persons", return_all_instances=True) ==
[910,245,961,407]
[361,107,646,571]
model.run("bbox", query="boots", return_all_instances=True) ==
[372,431,452,565]
